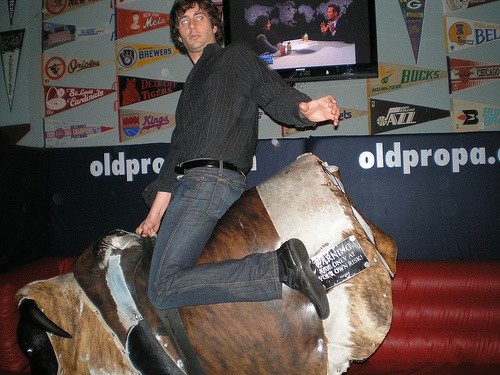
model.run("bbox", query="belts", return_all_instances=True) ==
[175,160,242,175]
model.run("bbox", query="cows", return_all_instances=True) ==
[14,152,398,375]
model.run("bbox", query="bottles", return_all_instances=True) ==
[279,40,286,56]
[303,33,309,43]
[285,41,292,55]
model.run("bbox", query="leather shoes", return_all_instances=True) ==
[277,238,330,320]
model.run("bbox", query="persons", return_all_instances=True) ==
[133,0,341,322]
[316,3,348,41]
[253,14,280,55]
